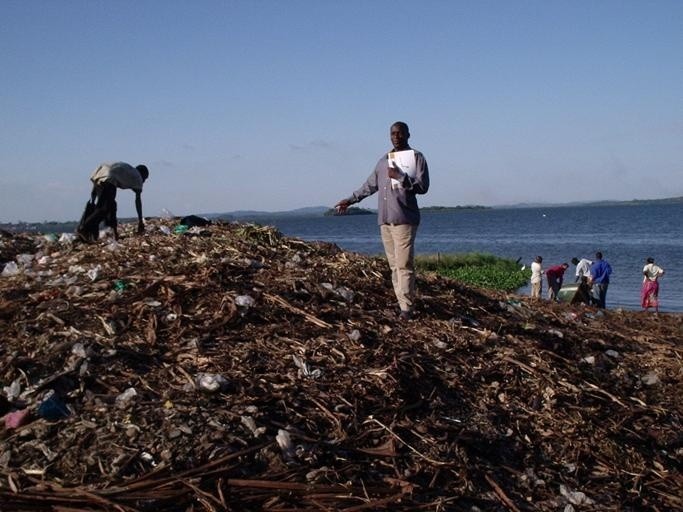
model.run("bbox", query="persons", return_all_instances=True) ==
[543,263,569,302]
[570,256,595,303]
[639,257,664,317]
[73,160,148,244]
[586,251,613,310]
[331,120,431,320]
[529,255,548,298]
[571,275,594,305]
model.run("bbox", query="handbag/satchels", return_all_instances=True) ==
[76,197,100,243]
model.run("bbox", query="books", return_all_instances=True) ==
[386,149,417,191]
